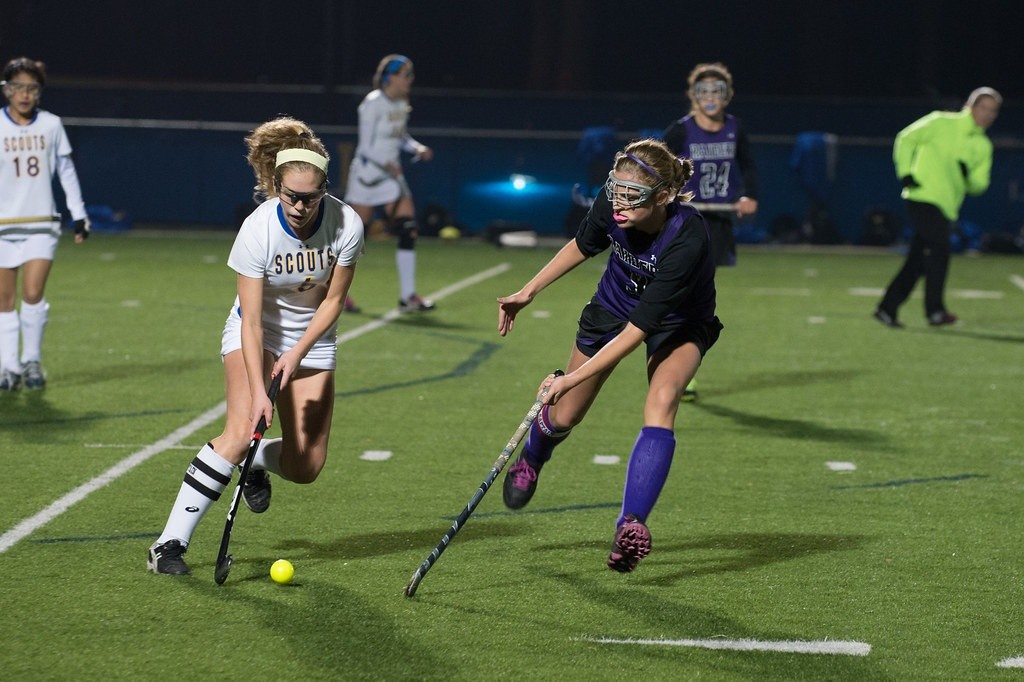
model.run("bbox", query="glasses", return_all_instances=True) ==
[277,187,326,207]
[603,172,653,209]
[696,82,724,101]
[9,81,40,96]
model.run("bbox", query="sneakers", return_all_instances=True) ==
[238,462,272,513]
[679,378,698,402]
[503,438,546,509]
[148,538,191,576]
[608,514,652,574]
[0,368,22,392]
[22,360,46,392]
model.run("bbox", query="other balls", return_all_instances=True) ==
[269,559,295,584]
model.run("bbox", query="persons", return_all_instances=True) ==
[496,140,724,573]
[342,54,437,317]
[144,118,364,576]
[872,85,1003,329]
[657,62,759,402]
[0,56,92,392]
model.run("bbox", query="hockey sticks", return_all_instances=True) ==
[214,371,286,585]
[358,158,423,188]
[406,369,565,599]
[0,216,64,224]
[571,183,739,213]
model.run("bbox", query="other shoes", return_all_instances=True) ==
[929,312,958,327]
[397,293,438,314]
[873,307,901,327]
[344,298,360,314]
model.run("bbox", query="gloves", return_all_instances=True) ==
[901,176,918,187]
[960,161,969,177]
